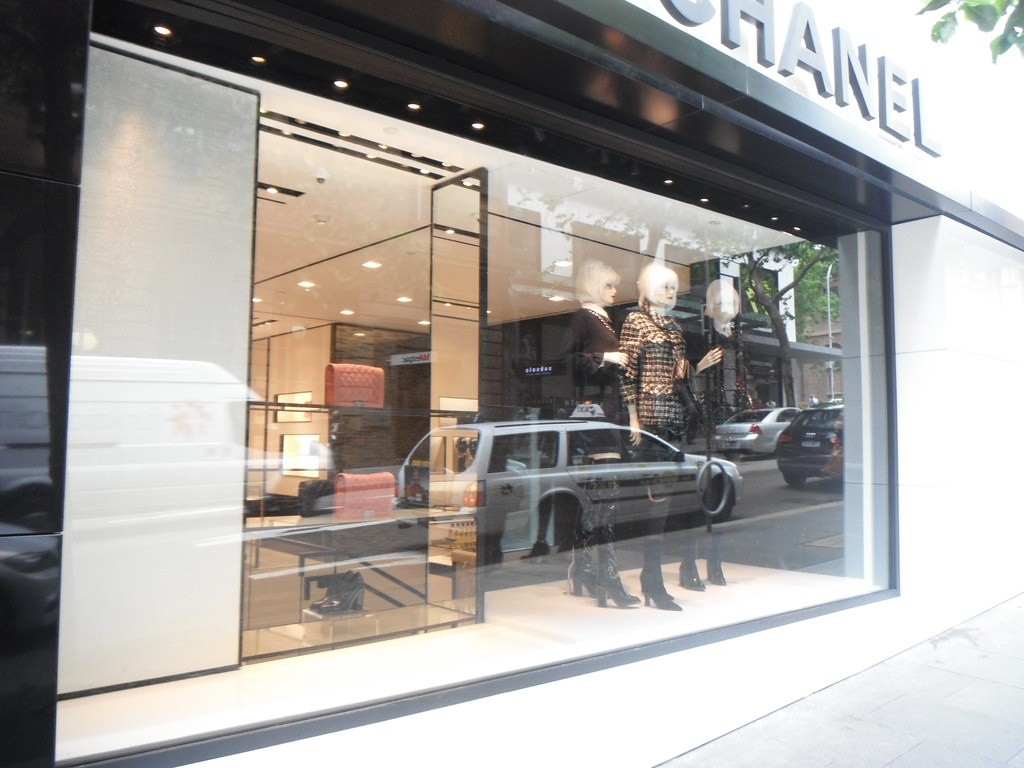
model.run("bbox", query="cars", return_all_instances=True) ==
[713,405,801,458]
[458,405,545,455]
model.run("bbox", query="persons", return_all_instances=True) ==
[568,260,640,608]
[616,265,687,611]
[680,279,746,590]
[809,396,818,406]
[766,399,777,408]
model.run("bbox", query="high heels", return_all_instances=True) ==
[310,571,365,615]
[520,540,550,565]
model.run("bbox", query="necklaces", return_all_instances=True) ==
[587,309,616,335]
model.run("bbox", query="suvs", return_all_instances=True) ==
[775,401,844,487]
[395,403,741,548]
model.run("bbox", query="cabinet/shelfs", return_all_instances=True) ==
[240,399,489,664]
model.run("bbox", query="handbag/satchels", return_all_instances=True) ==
[334,472,395,521]
[672,359,704,418]
[325,363,384,409]
[298,480,336,518]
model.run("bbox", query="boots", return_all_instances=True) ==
[706,530,727,585]
[594,458,641,608]
[679,535,706,590]
[639,539,683,612]
[569,457,597,597]
